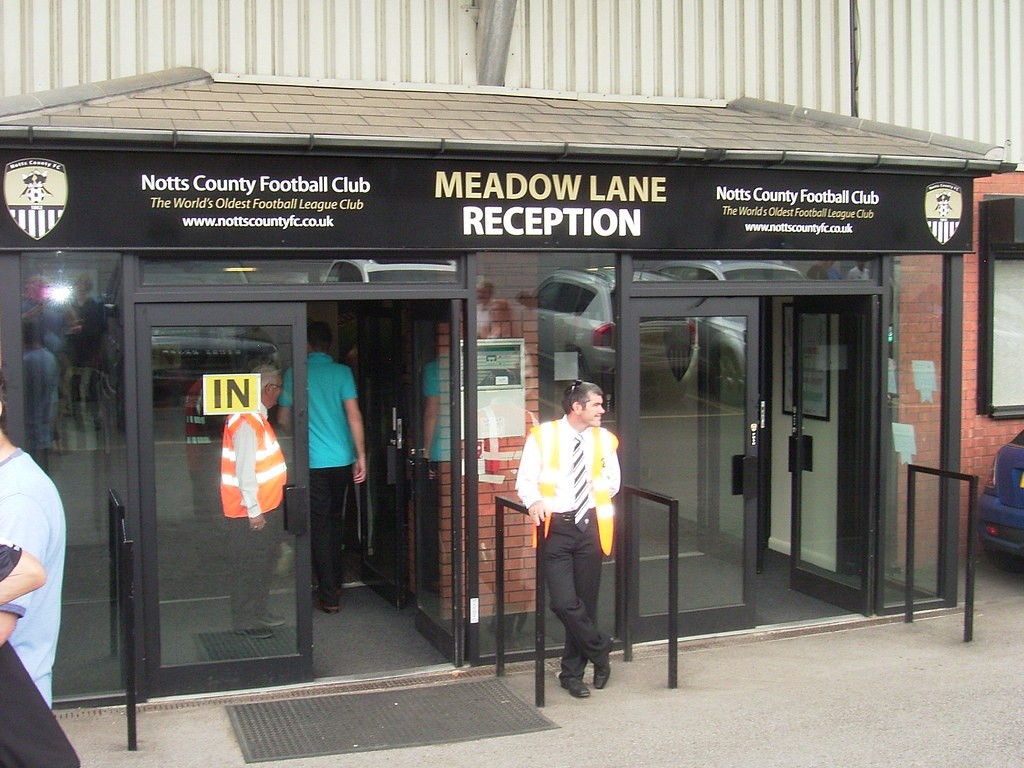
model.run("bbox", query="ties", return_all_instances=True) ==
[573,435,591,533]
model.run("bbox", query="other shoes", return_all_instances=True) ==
[312,590,339,614]
[235,624,273,638]
[257,613,286,626]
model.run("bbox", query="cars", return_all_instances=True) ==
[974,427,1023,571]
[650,260,810,406]
[103,258,278,403]
[324,260,457,284]
[521,266,698,393]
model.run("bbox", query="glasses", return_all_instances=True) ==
[265,383,283,389]
[568,380,582,411]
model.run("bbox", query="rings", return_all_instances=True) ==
[533,511,538,515]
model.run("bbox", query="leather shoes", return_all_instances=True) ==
[593,637,614,688]
[561,681,590,697]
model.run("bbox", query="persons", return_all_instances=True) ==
[0,404,67,709]
[420,342,459,629]
[218,362,291,639]
[279,322,368,615]
[804,260,876,281]
[0,536,47,607]
[11,272,258,579]
[472,276,518,339]
[514,379,621,698]
[460,369,540,635]
